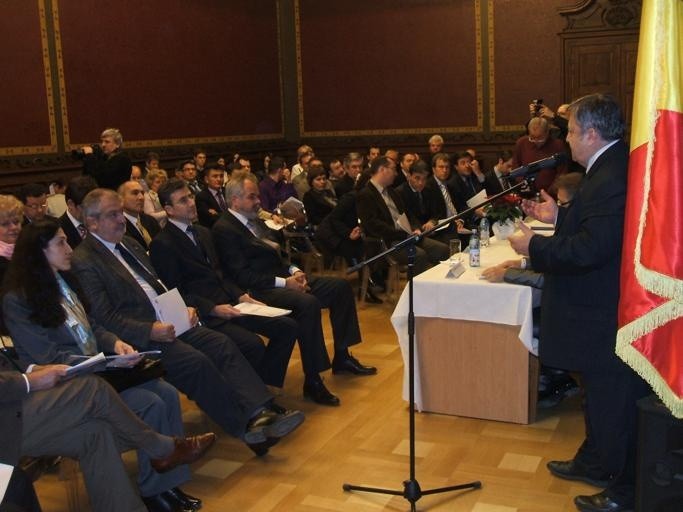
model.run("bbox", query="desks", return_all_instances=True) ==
[389,220,557,424]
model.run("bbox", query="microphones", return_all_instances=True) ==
[509,152,567,178]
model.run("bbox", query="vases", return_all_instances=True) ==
[492,218,516,241]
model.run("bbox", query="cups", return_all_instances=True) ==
[449,239,463,266]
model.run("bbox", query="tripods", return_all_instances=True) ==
[343,178,535,512]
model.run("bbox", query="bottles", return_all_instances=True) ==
[478,212,490,247]
[535,192,541,204]
[514,200,522,228]
[468,228,481,268]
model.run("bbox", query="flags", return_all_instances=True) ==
[614,1,683,421]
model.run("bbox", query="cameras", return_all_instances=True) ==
[71,145,100,161]
[534,99,543,112]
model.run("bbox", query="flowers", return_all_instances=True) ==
[482,194,524,224]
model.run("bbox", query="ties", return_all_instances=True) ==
[440,184,458,216]
[417,191,425,215]
[135,217,153,249]
[187,224,209,266]
[189,182,200,195]
[382,190,402,231]
[77,223,89,240]
[465,176,475,195]
[115,242,167,298]
[501,175,510,191]
[247,222,282,260]
[216,191,227,213]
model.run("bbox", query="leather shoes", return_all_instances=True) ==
[573,489,629,512]
[546,458,612,488]
[147,431,218,475]
[302,379,341,407]
[139,486,202,512]
[17,456,68,484]
[242,401,306,457]
[537,373,581,410]
[330,354,378,376]
[357,288,384,305]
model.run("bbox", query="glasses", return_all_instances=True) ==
[557,199,573,208]
[23,200,49,210]
[529,135,547,144]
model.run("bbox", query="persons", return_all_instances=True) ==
[0,91,683,512]
